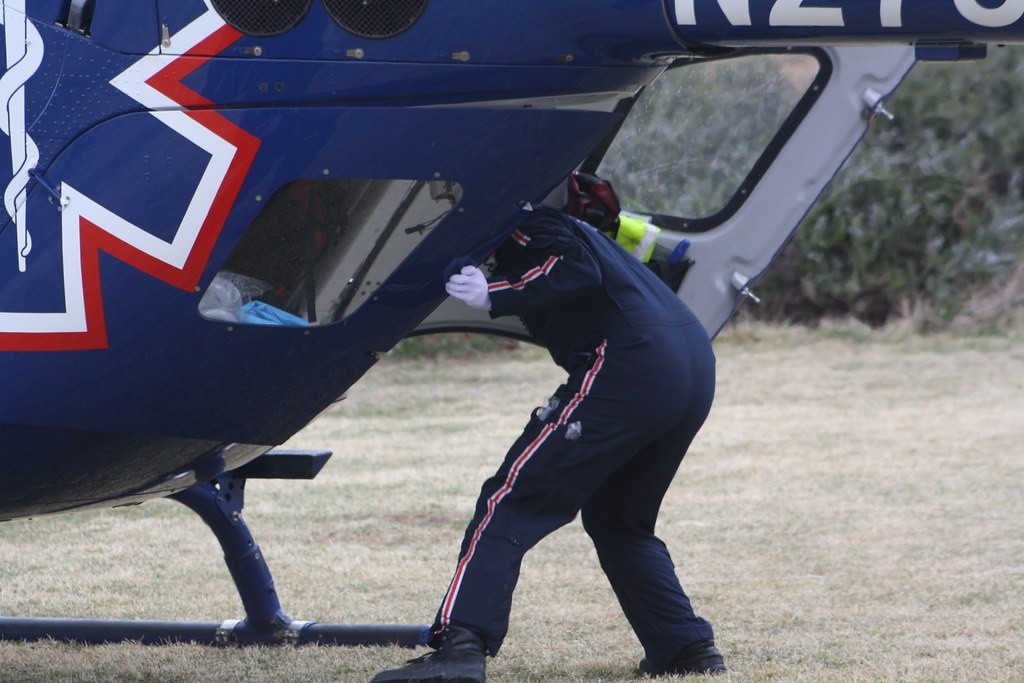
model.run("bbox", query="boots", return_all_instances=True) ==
[640,641,727,676]
[371,626,487,682]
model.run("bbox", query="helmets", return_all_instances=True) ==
[402,180,464,234]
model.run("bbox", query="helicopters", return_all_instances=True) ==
[1,0,1024,646]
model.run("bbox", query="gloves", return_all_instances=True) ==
[447,265,492,313]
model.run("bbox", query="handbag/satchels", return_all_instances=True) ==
[564,168,621,231]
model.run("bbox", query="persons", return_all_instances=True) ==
[370,180,726,683]
